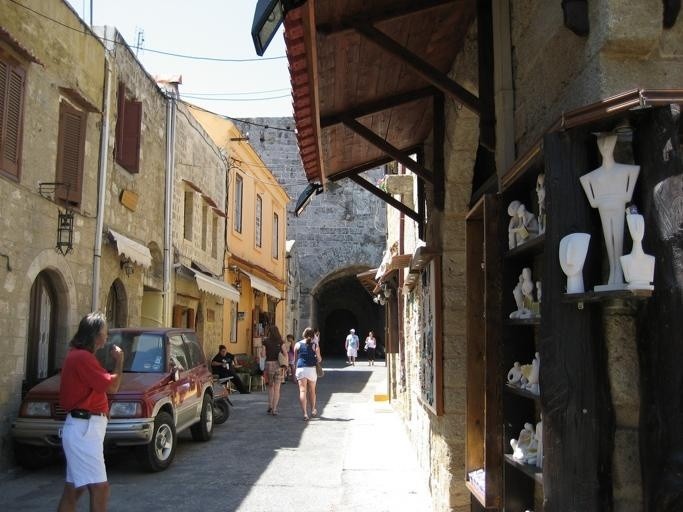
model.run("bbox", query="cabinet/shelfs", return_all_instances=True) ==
[464,195,502,512]
[502,125,613,512]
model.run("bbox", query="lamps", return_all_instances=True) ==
[223,265,242,295]
[39,182,75,256]
[120,260,134,275]
[251,0,287,56]
[295,183,323,217]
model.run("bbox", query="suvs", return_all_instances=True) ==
[8,325,215,471]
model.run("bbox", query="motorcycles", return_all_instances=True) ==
[212,374,234,425]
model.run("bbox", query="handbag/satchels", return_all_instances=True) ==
[316,363,324,378]
[278,352,289,368]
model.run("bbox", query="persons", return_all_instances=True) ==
[210,345,251,394]
[364,329,376,366]
[345,329,359,366]
[56,310,124,512]
[286,327,324,421]
[261,326,287,416]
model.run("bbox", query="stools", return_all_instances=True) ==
[248,375,267,392]
